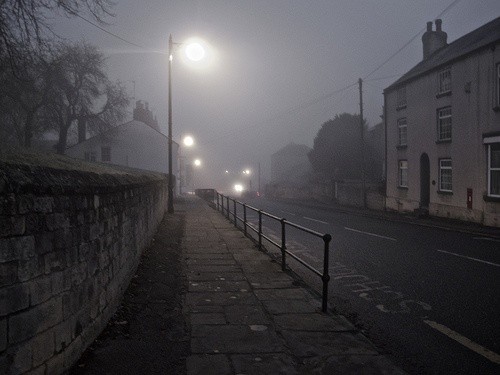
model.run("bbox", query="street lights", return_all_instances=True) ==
[168,34,207,214]
